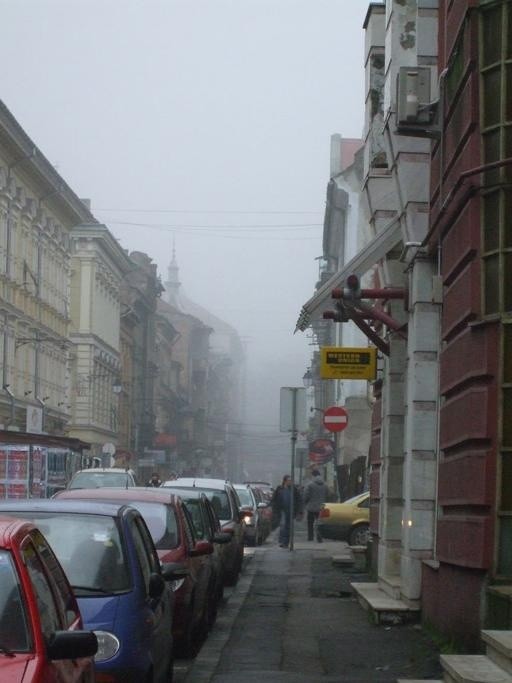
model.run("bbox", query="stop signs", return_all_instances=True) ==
[323,406,349,433]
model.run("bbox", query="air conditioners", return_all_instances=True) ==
[396,66,433,124]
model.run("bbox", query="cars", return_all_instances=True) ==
[315,489,370,547]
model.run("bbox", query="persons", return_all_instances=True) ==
[303,470,329,543]
[149,473,161,487]
[272,475,300,547]
